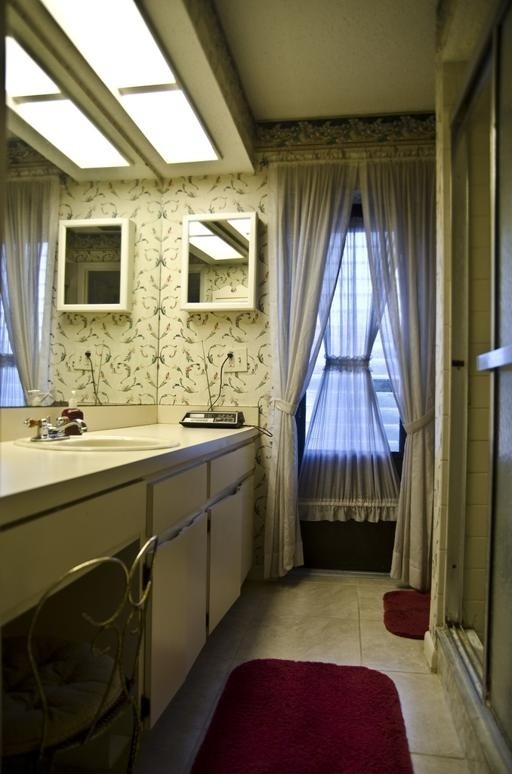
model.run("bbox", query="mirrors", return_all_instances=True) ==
[181,211,257,311]
[0,128,163,408]
[56,218,134,314]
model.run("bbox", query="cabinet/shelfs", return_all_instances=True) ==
[208,434,262,637]
[0,455,209,730]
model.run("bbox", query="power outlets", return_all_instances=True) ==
[223,347,248,373]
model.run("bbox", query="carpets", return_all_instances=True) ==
[189,657,413,773]
[383,589,430,638]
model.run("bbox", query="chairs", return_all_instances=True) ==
[0,533,159,773]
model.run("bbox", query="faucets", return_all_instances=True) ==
[57,418,88,435]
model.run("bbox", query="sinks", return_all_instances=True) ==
[16,436,180,451]
